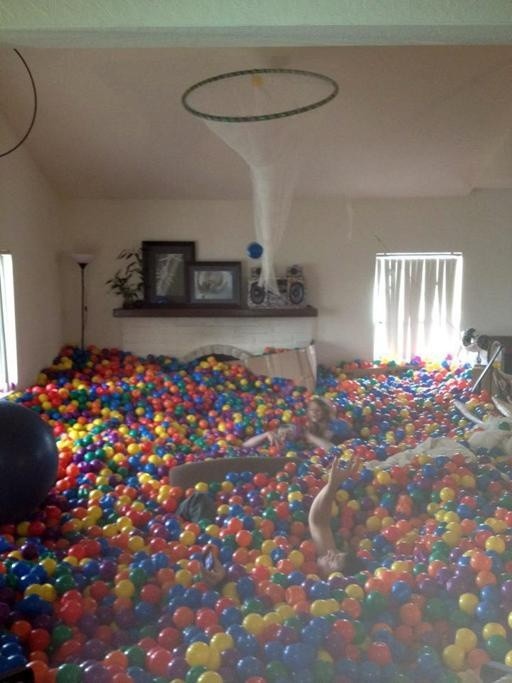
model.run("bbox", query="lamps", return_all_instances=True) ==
[71,254,97,350]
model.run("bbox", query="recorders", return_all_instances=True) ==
[247,277,306,309]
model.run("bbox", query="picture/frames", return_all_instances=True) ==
[184,262,242,307]
[141,239,196,305]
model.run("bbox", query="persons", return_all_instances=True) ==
[243,395,355,451]
[201,555,229,593]
[176,492,218,524]
[309,455,367,577]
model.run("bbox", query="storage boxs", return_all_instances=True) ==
[247,345,316,392]
